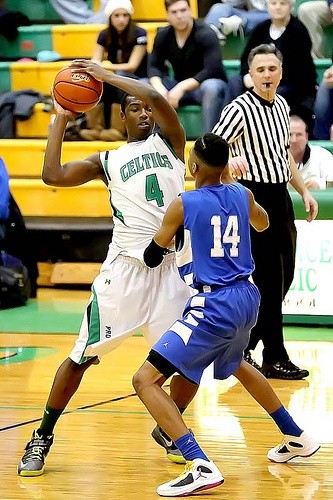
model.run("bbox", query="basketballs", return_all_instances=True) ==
[53,64,103,112]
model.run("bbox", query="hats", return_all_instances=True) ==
[105,0,135,17]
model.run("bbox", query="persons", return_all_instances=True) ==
[133,132,321,497]
[203,45,320,381]
[74,2,333,194]
[18,61,200,479]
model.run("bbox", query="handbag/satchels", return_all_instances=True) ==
[0,264,29,310]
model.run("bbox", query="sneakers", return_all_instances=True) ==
[261,360,309,380]
[244,352,260,370]
[17,430,54,476]
[151,424,187,463]
[156,458,225,496]
[267,429,322,462]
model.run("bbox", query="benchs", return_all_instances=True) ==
[0,0,333,285]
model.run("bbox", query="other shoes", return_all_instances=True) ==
[78,124,105,140]
[99,127,125,140]
